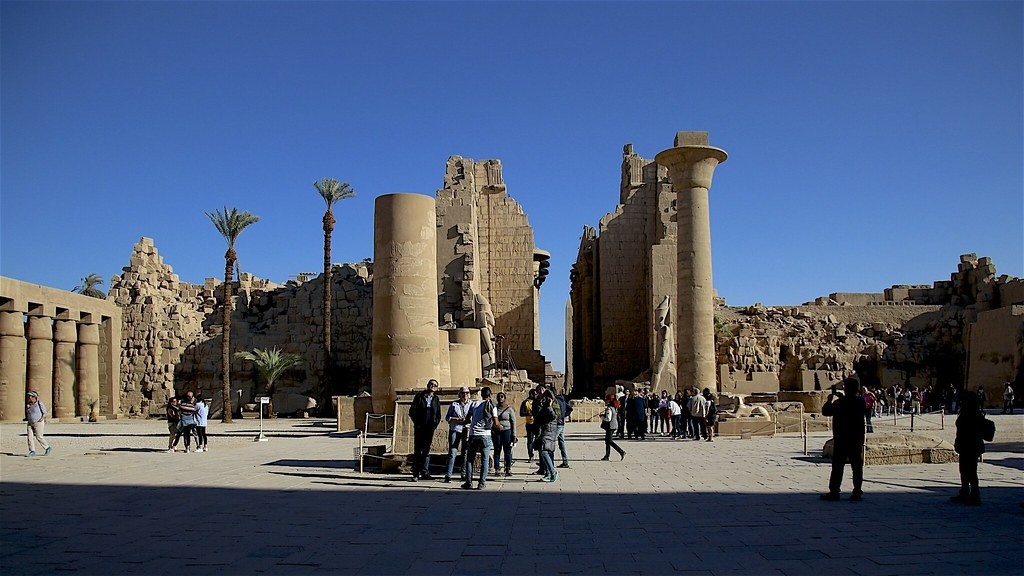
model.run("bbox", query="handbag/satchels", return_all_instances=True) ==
[601,420,611,431]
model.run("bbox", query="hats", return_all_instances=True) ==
[28,392,37,397]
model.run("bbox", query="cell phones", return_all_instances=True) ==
[832,385,836,395]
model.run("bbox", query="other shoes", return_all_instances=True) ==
[505,472,512,476]
[495,471,501,477]
[615,431,713,443]
[445,477,451,483]
[536,463,540,466]
[820,491,840,500]
[601,457,609,461]
[966,491,979,504]
[533,470,546,474]
[850,494,861,501]
[44,448,51,456]
[621,451,627,461]
[528,457,533,463]
[461,483,473,488]
[423,475,435,480]
[951,493,968,500]
[167,445,208,453]
[478,483,486,489]
[557,463,569,468]
[412,475,419,481]
[26,452,35,456]
[460,476,466,481]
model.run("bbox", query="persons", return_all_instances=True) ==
[26,391,51,457]
[1000,381,1014,415]
[490,392,517,477]
[866,384,935,416]
[460,387,504,490]
[821,378,867,502]
[614,386,718,443]
[520,384,574,482]
[167,390,209,453]
[947,384,957,413]
[977,385,986,412]
[599,394,627,461]
[445,387,477,484]
[948,390,985,506]
[860,386,877,434]
[409,379,441,481]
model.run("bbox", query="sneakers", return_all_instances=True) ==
[551,472,557,482]
[540,476,551,482]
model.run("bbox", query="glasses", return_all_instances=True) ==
[431,383,438,387]
[461,392,469,394]
